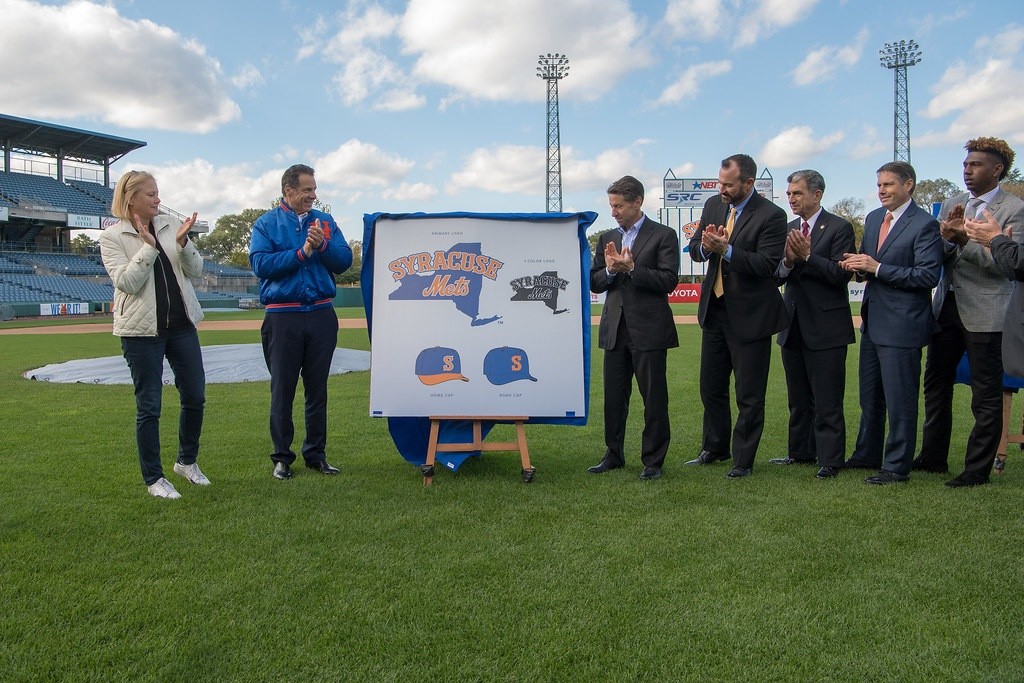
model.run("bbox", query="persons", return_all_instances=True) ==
[913,136,1024,487]
[589,175,680,480]
[838,161,944,485]
[99,170,210,499]
[769,169,857,480]
[684,154,789,480]
[249,164,353,480]
[963,209,1024,283]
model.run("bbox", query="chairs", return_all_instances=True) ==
[0,252,114,303]
[0,170,115,210]
[203,258,254,279]
[195,288,260,302]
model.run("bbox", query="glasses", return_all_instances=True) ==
[122,170,138,194]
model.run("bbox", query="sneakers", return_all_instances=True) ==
[173,461,211,486]
[148,477,181,499]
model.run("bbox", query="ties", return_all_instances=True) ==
[713,208,736,298]
[802,222,809,239]
[877,213,892,254]
[964,199,984,221]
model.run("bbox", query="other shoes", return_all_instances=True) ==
[943,471,990,488]
[911,452,949,474]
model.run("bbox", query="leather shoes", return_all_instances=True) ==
[587,458,623,473]
[768,456,817,465]
[725,466,753,478]
[864,469,910,485]
[815,466,840,480]
[306,460,340,475]
[843,451,882,469]
[639,466,661,481]
[684,449,732,466]
[273,462,293,480]
